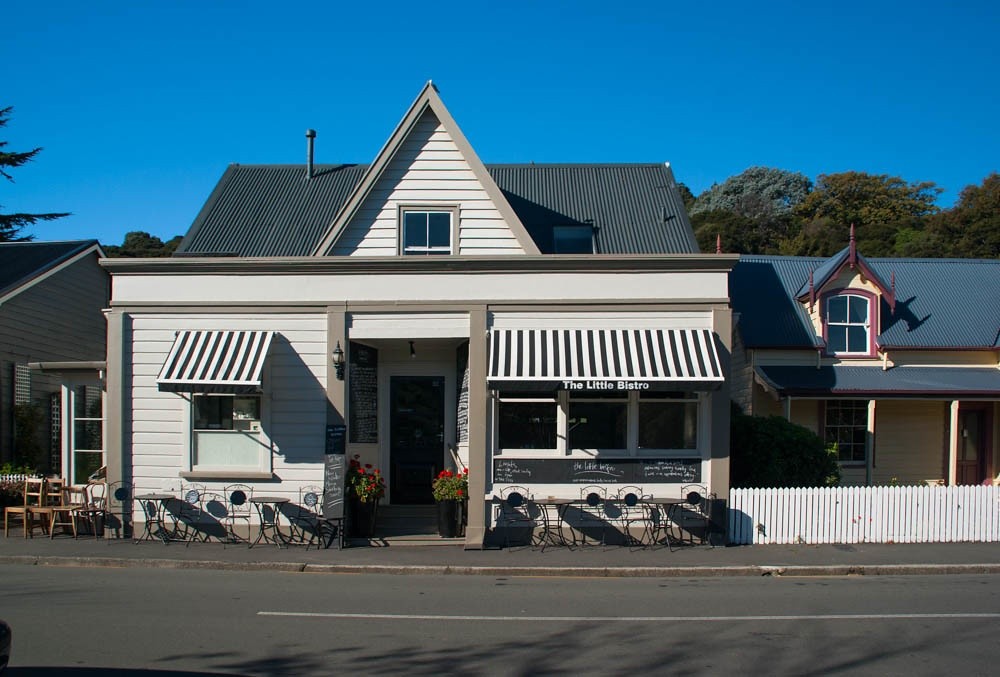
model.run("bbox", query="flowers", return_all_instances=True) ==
[345,455,386,504]
[431,466,470,502]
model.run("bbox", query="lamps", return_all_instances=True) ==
[409,341,415,358]
[331,340,347,380]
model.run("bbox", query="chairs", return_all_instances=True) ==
[3,476,345,551]
[494,484,717,552]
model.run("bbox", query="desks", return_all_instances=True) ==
[530,498,575,552]
[249,497,289,548]
[134,493,173,546]
[642,497,686,552]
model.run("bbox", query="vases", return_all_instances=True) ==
[351,496,375,537]
[436,501,463,537]
